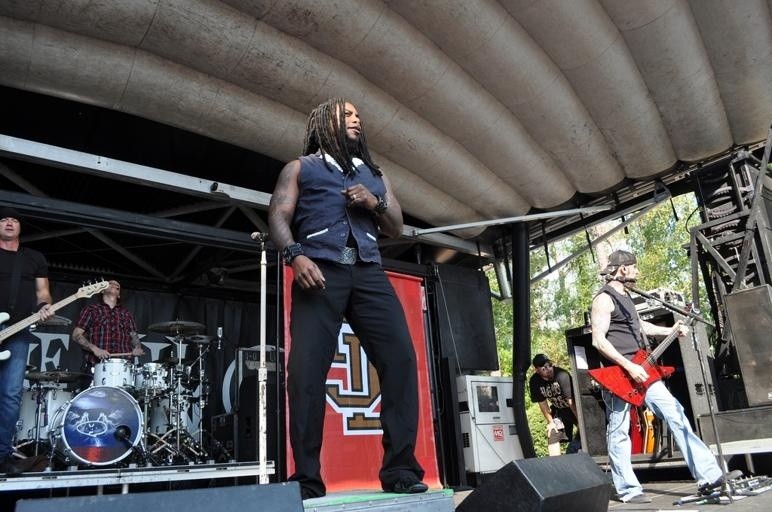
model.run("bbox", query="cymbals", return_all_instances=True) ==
[148,321,204,335]
[26,371,93,382]
[186,335,213,343]
[40,314,71,326]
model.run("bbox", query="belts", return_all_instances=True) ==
[338,246,375,267]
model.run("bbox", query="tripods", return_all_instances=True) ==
[626,286,758,506]
[142,365,234,466]
[13,390,49,470]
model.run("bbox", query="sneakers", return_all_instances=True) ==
[697,475,732,498]
[628,494,652,503]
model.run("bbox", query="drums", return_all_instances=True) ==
[15,387,73,443]
[138,363,168,396]
[48,385,142,464]
[92,359,136,391]
[151,394,188,438]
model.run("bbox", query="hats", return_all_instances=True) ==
[600,250,636,276]
[533,353,554,368]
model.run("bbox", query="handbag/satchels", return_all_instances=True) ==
[13,454,50,473]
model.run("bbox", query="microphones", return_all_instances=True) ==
[216,320,224,351]
[250,232,272,242]
[604,274,637,284]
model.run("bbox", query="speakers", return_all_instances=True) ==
[723,284,772,406]
[454,452,611,512]
[15,481,306,512]
[430,262,499,371]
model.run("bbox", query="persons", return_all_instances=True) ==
[529,352,581,455]
[1,206,55,476]
[266,95,428,503]
[72,279,144,380]
[588,249,742,502]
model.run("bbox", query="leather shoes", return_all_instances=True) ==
[382,470,428,493]
[0,459,24,476]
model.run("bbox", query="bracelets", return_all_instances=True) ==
[281,243,305,266]
[369,191,387,216]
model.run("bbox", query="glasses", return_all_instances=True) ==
[538,363,552,371]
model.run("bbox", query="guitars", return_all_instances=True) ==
[587,303,700,407]
[1,277,108,361]
